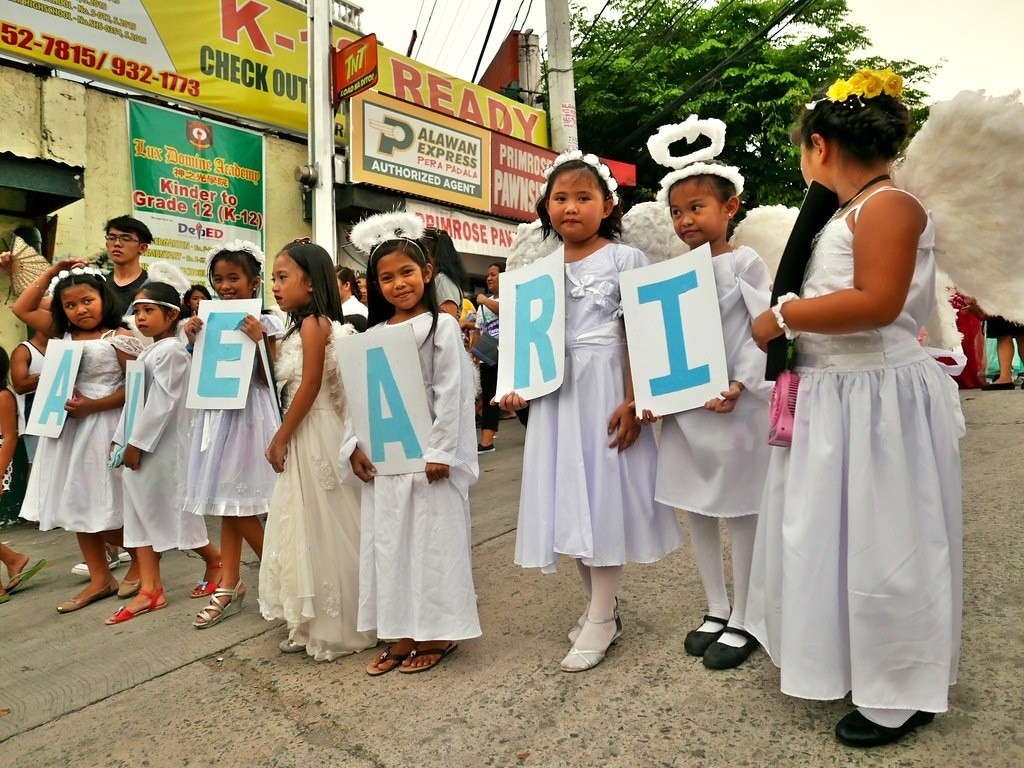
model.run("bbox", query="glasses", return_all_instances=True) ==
[104,234,144,243]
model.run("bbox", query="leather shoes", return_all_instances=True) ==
[835,710,936,748]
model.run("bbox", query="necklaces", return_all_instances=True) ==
[798,185,868,297]
[809,174,891,246]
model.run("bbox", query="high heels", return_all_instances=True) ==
[191,578,246,629]
[559,596,623,672]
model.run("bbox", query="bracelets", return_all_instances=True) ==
[32,279,46,293]
[770,292,802,340]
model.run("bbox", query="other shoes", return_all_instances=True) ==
[279,640,306,653]
[500,413,517,420]
[56,576,120,613]
[684,605,732,656]
[478,443,496,455]
[116,552,162,600]
[703,627,760,670]
[981,382,1016,391]
[118,551,132,562]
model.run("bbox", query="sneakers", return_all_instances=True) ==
[71,547,120,575]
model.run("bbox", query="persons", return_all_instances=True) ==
[0,67,1024,748]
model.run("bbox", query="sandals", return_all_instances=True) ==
[191,562,223,598]
[105,588,168,625]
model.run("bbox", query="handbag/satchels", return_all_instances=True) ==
[469,332,499,367]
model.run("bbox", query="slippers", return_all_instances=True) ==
[3,559,47,595]
[366,646,413,676]
[0,594,10,604]
[399,641,459,674]
